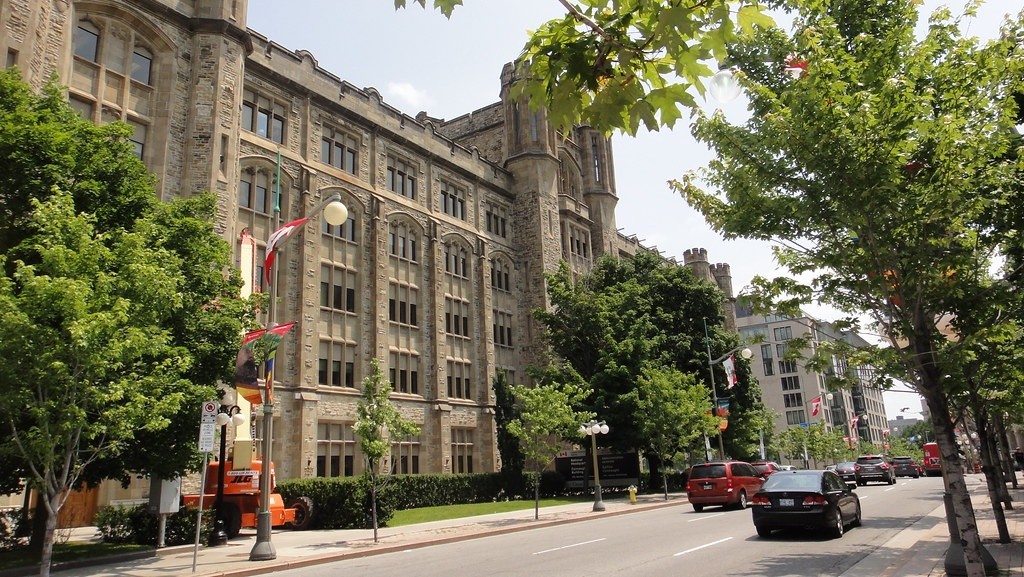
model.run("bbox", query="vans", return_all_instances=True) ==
[854,436,896,486]
[752,461,781,479]
[687,460,766,512]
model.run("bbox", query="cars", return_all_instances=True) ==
[751,468,861,539]
[825,462,857,485]
[892,457,924,478]
[989,448,1020,472]
[780,465,797,471]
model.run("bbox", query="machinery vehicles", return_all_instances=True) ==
[181,438,314,540]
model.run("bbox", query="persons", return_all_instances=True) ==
[1015,446,1024,471]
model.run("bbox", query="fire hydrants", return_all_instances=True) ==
[627,483,638,505]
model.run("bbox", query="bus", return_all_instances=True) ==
[922,442,965,477]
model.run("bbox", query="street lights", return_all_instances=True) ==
[250,191,349,561]
[703,316,751,460]
[205,395,244,547]
[819,369,834,432]
[577,419,609,511]
[847,406,868,452]
[882,426,898,452]
[908,433,921,450]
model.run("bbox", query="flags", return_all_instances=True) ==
[883,430,889,437]
[851,416,859,428]
[265,219,306,286]
[812,396,820,416]
[722,354,737,387]
